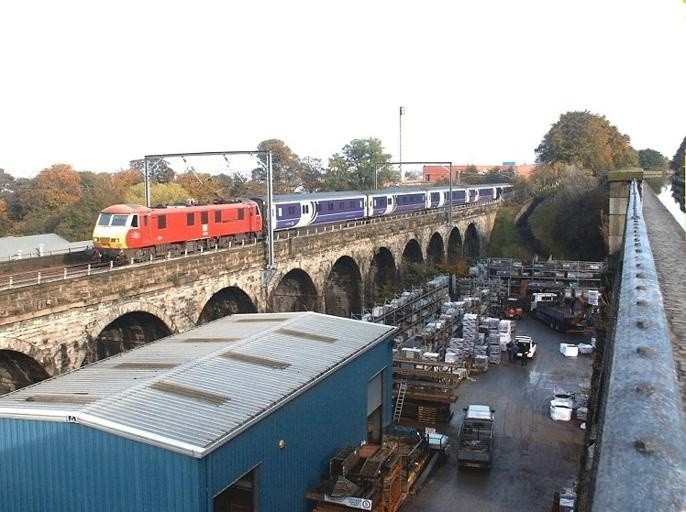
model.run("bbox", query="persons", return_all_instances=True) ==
[513,341,519,358]
[506,341,514,360]
[521,343,529,367]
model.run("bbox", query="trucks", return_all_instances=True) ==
[531,293,556,312]
[515,336,537,359]
[455,404,496,471]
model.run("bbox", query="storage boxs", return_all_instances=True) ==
[425,290,513,374]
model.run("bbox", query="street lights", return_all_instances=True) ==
[400,108,405,180]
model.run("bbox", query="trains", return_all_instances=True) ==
[92,182,514,266]
